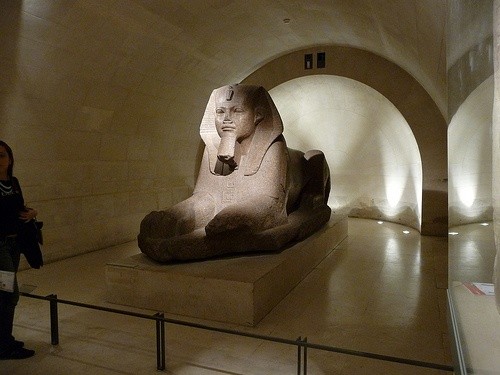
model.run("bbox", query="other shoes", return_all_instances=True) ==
[1,339,35,360]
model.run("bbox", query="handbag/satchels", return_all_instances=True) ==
[21,218,46,270]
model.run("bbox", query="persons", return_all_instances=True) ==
[0,141,38,360]
[139,84,332,242]
